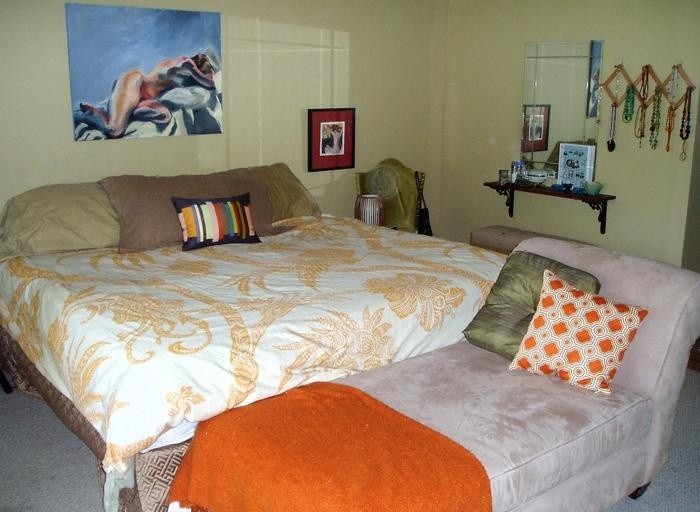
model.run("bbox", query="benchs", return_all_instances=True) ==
[470,224,598,264]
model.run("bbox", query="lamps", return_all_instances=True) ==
[354,195,384,227]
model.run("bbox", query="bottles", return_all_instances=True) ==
[511,161,521,183]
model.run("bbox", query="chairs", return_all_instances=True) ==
[356,158,425,236]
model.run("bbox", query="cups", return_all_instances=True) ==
[584,182,604,196]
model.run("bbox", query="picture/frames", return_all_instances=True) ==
[558,142,591,183]
[524,104,551,152]
[307,108,355,172]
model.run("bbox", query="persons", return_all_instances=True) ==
[321,123,343,155]
[80,43,221,138]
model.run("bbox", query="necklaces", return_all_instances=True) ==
[604,62,691,161]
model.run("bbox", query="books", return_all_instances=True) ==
[557,143,595,185]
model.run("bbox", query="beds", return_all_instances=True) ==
[0,214,508,512]
[165,235,700,512]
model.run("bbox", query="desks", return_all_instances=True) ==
[484,180,616,234]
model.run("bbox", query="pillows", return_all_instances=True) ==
[98,166,275,255]
[171,192,263,251]
[464,251,600,363]
[507,268,649,395]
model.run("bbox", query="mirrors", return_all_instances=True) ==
[520,40,604,172]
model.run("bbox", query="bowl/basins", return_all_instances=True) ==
[528,169,546,185]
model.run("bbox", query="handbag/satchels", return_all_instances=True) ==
[415,170,435,235]
